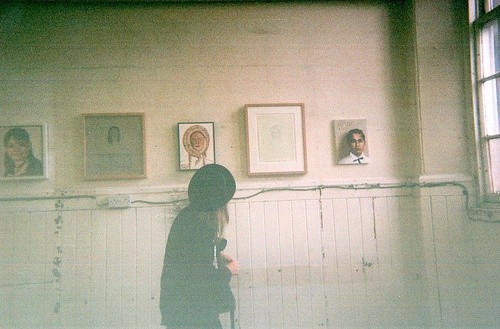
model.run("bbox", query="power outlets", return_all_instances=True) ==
[108,195,131,209]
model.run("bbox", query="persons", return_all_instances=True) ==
[162,163,241,329]
[0,126,43,175]
[335,122,369,165]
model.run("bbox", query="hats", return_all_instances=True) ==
[188,164,236,210]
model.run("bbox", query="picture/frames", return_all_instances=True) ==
[178,122,215,171]
[244,103,308,176]
[0,123,49,180]
[80,112,147,181]
